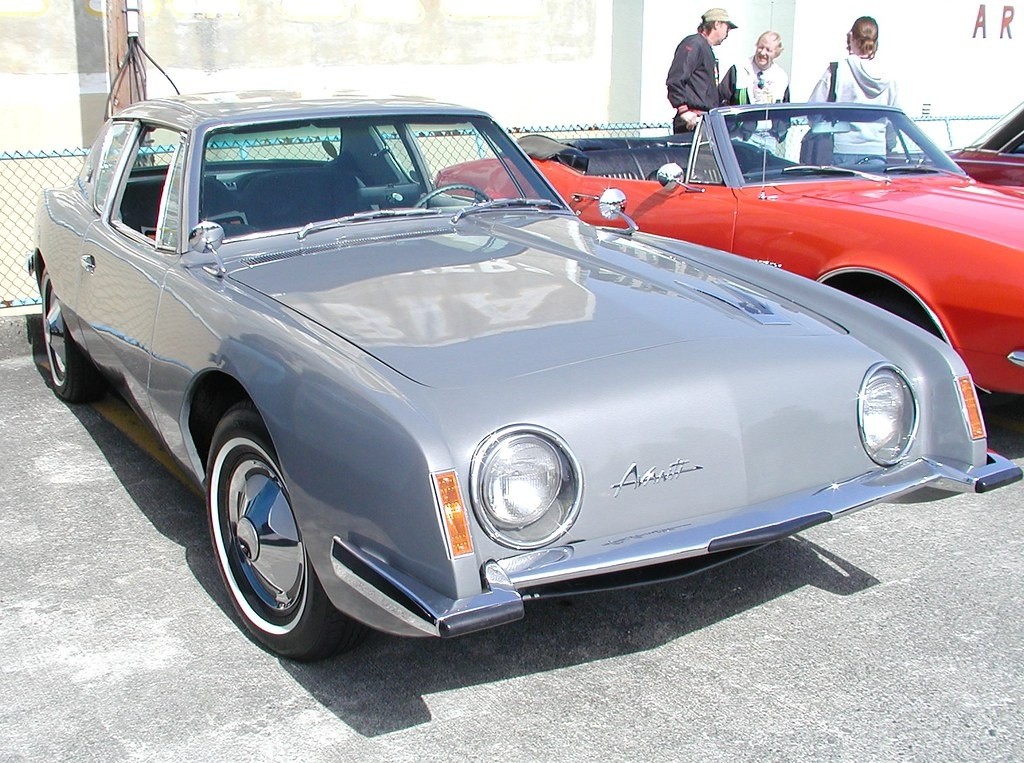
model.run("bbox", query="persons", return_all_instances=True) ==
[717,29,794,157]
[666,6,738,135]
[805,16,903,173]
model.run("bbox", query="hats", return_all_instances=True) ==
[699,6,739,30]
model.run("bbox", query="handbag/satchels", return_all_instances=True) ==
[798,130,835,168]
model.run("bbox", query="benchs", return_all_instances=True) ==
[233,167,358,225]
[516,131,778,183]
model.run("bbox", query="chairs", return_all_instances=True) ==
[146,174,241,226]
[359,181,423,211]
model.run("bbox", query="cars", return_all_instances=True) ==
[885,97,1024,191]
[424,96,1024,408]
[33,90,1024,672]
